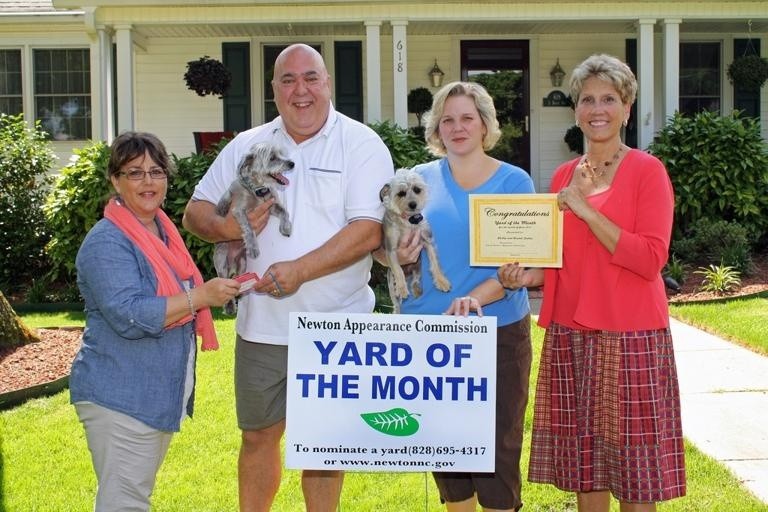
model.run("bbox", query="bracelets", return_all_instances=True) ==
[186,289,197,315]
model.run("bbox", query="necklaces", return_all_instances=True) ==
[580,140,624,191]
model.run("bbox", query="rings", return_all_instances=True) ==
[274,289,280,298]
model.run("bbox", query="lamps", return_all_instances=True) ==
[550,56,566,87]
[428,58,444,87]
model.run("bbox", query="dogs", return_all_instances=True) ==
[379,166,453,314]
[212,138,296,317]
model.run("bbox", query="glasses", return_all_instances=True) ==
[120,168,168,179]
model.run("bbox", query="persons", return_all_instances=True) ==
[494,48,689,511]
[179,41,397,511]
[64,129,244,511]
[374,78,541,512]
